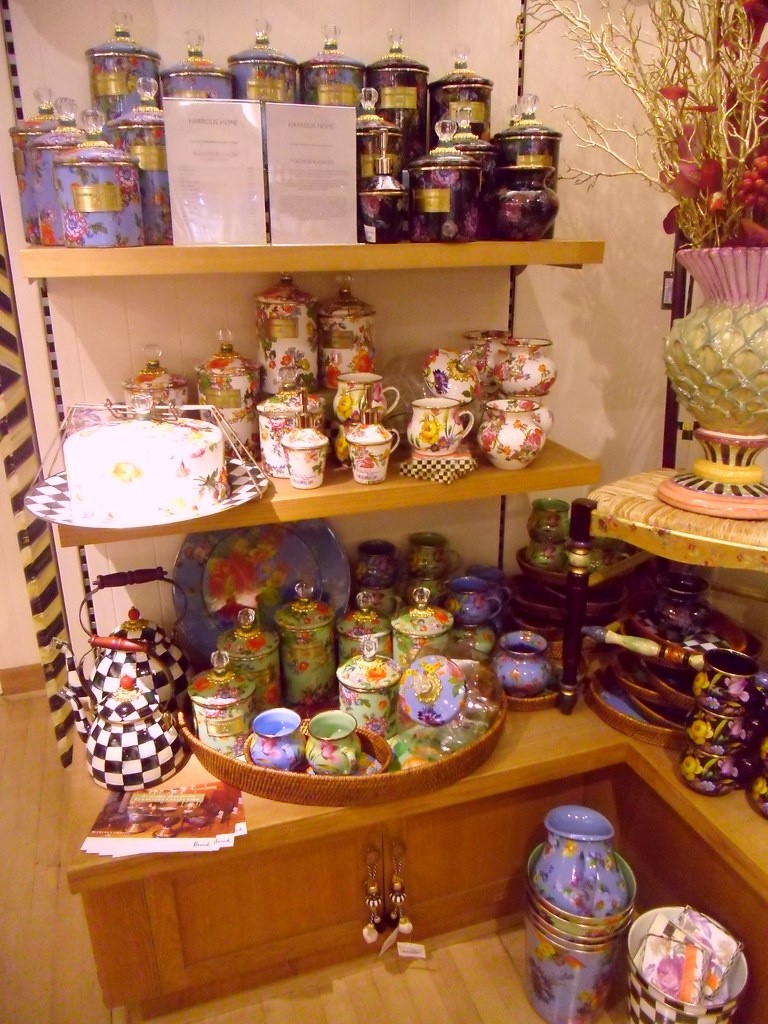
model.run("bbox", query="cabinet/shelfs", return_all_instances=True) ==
[21,240,607,547]
[76,762,768,1024]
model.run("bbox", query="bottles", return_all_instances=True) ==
[352,532,554,697]
[250,707,362,776]
[406,328,559,472]
[531,803,630,918]
[679,648,768,820]
[120,272,400,491]
[8,24,560,250]
[525,497,572,572]
[186,531,455,758]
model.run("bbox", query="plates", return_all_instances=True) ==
[169,519,350,660]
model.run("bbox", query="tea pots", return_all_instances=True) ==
[59,567,198,795]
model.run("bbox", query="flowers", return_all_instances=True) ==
[510,0,768,248]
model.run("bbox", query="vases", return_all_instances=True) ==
[653,246,767,521]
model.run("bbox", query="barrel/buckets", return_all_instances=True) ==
[521,841,749,1024]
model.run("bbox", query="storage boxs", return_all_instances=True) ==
[161,97,268,245]
[265,101,359,244]
